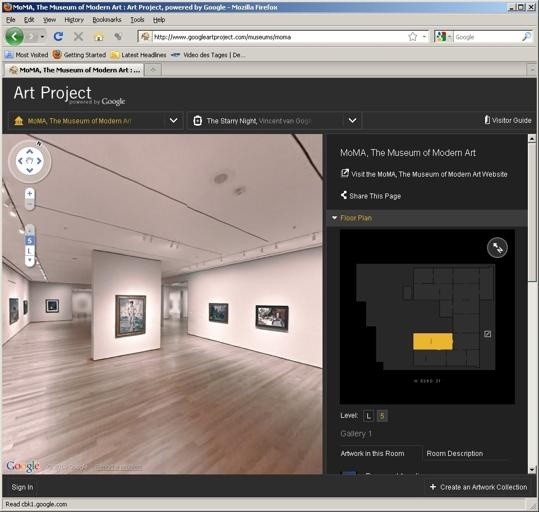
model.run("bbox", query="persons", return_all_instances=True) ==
[271,311,285,327]
[126,300,138,332]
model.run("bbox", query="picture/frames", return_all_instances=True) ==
[115,294,146,337]
[209,303,228,324]
[256,304,288,333]
[9,298,18,325]
[46,299,59,313]
